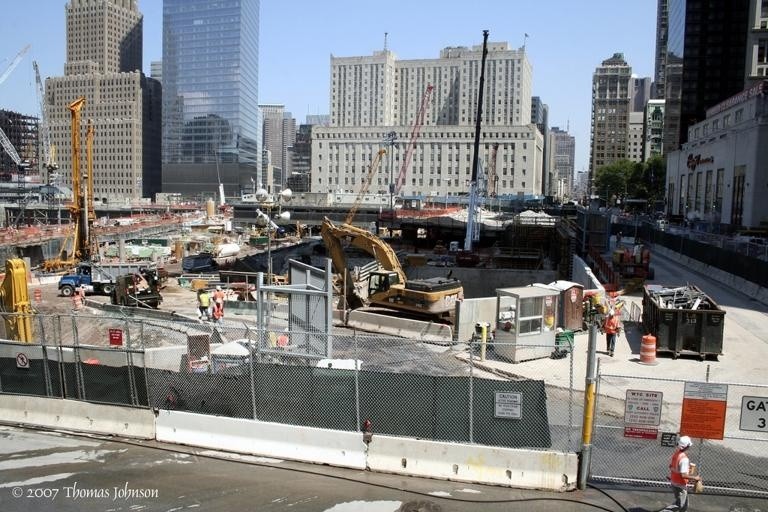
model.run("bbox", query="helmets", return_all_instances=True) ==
[678,436,693,447]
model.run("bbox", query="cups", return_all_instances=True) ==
[690,463,695,474]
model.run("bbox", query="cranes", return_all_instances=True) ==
[345,149,387,225]
[0,40,57,227]
[395,81,434,194]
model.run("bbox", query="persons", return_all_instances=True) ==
[623,240,651,266]
[212,302,223,324]
[657,435,702,512]
[197,288,210,321]
[604,310,621,357]
[614,231,625,251]
[212,285,224,308]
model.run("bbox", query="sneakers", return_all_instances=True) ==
[607,352,614,357]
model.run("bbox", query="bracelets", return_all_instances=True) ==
[692,475,695,479]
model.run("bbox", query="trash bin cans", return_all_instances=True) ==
[475,323,490,341]
[449,242,459,252]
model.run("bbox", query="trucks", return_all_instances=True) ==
[57,261,159,297]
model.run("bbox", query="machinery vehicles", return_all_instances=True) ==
[109,269,163,309]
[320,215,464,326]
